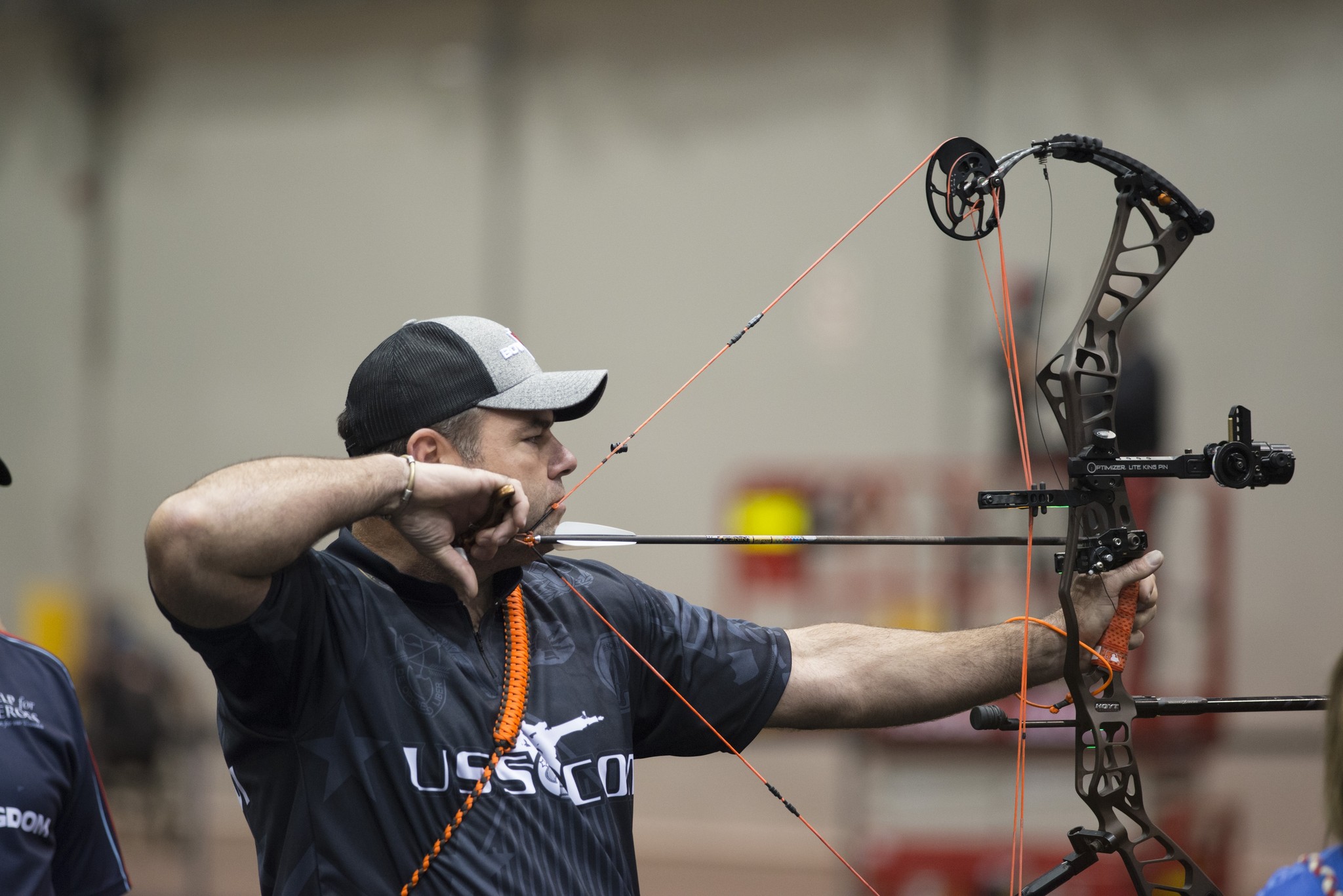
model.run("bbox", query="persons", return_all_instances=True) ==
[1255,652,1343,896]
[0,459,133,896]
[142,315,1166,896]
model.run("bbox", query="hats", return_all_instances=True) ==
[345,315,608,458]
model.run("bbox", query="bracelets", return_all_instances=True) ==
[382,453,416,520]
[1000,616,1112,716]
